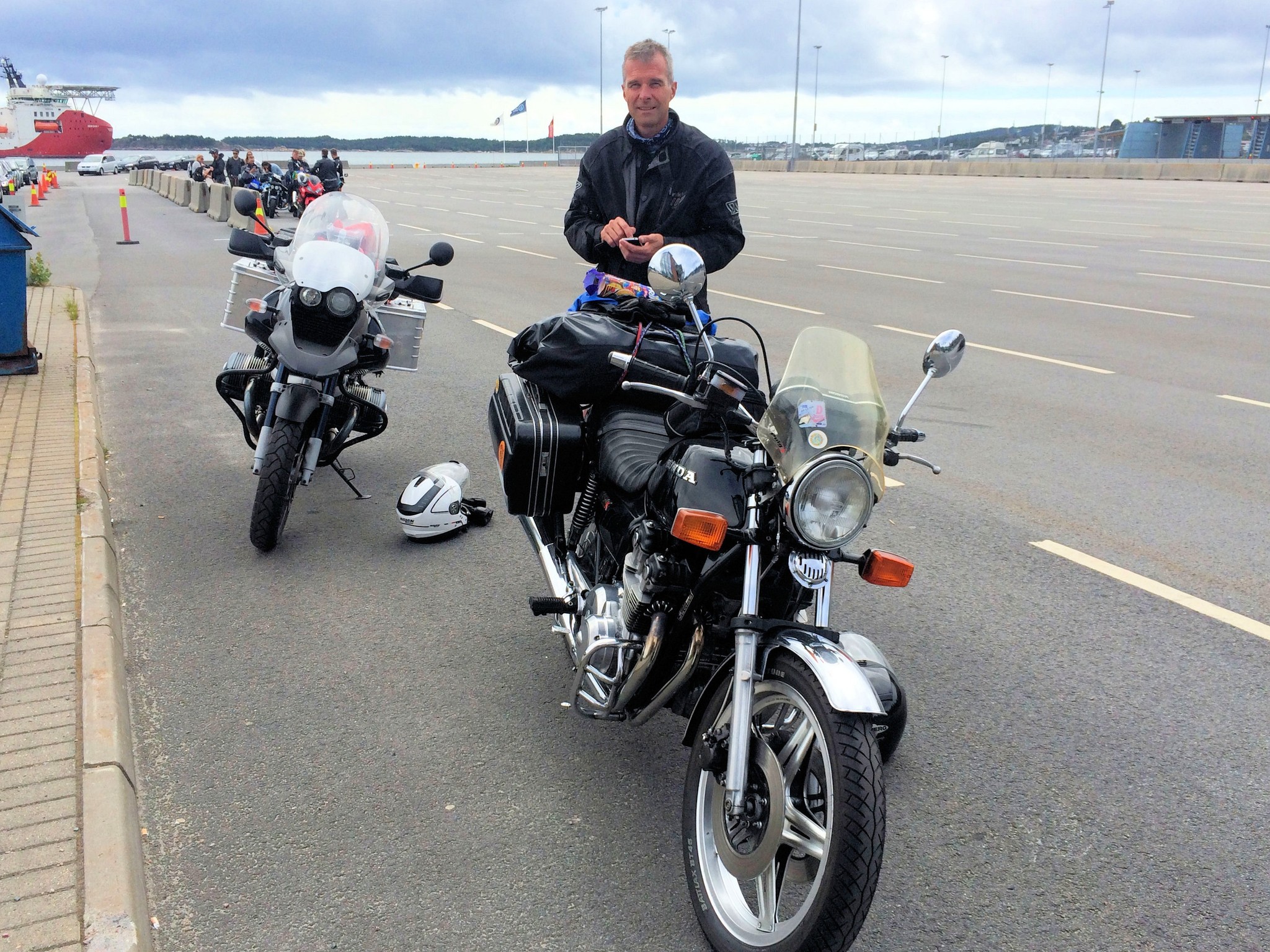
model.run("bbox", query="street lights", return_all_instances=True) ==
[935,54,950,152]
[1254,23,1270,113]
[661,29,677,51]
[812,45,822,149]
[593,5,608,137]
[1037,63,1055,158]
[1092,0,1115,158]
[1129,70,1141,124]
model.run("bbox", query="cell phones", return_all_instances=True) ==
[625,238,643,246]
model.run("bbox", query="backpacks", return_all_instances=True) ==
[193,166,207,181]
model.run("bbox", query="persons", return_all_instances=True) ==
[191,148,345,194]
[564,39,745,314]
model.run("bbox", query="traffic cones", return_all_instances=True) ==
[27,183,43,207]
[253,197,271,235]
[40,174,51,193]
[368,161,547,169]
[41,163,47,172]
[51,169,61,189]
[37,181,48,200]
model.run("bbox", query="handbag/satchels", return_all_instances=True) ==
[569,291,717,337]
[506,311,758,407]
[656,389,768,466]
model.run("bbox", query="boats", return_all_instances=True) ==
[0,54,122,161]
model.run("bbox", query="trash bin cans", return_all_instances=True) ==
[0,203,43,375]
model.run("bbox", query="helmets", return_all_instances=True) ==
[396,471,470,544]
[232,147,239,152]
[209,147,219,153]
[299,151,304,156]
[243,173,253,184]
[838,633,907,764]
[297,172,309,187]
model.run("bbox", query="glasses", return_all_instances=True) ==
[248,154,251,156]
[233,152,238,155]
[200,159,204,160]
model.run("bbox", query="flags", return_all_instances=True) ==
[490,113,503,126]
[510,101,526,117]
[548,120,554,138]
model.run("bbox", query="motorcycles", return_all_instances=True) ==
[484,240,967,952]
[212,190,459,555]
[262,173,325,219]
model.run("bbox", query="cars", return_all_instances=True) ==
[725,139,1121,161]
[117,154,159,173]
[77,153,119,176]
[0,156,39,204]
[158,154,228,171]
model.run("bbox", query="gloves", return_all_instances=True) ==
[461,497,493,527]
[606,295,685,331]
